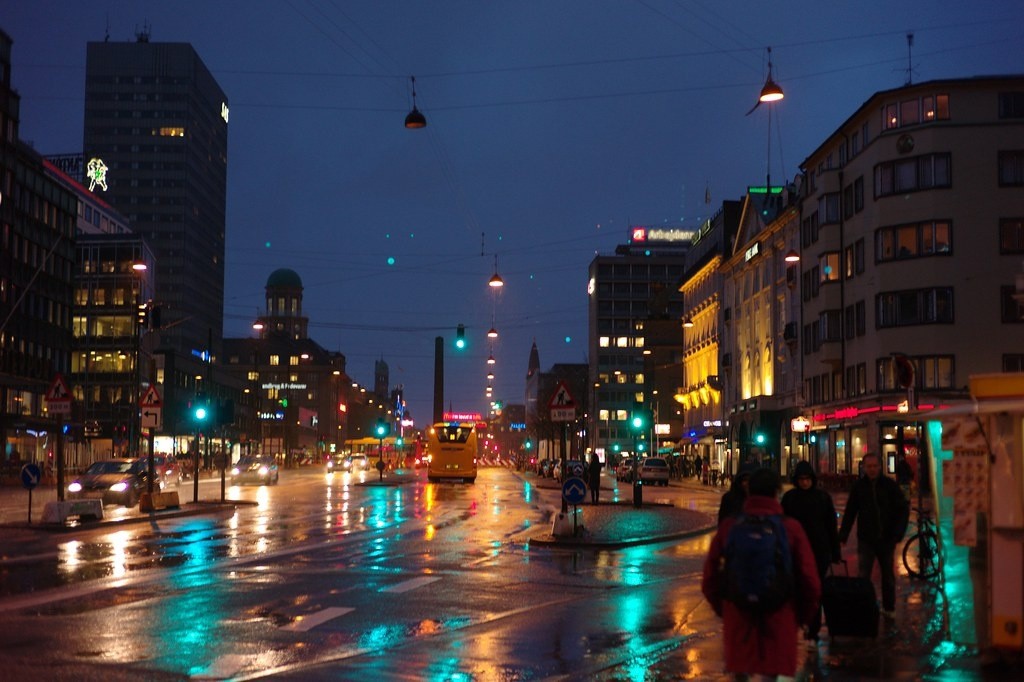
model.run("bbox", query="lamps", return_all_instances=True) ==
[486,391,491,397]
[760,45,785,102]
[488,254,504,287]
[487,314,498,337]
[487,352,496,364]
[487,369,494,379]
[404,76,427,129]
[785,230,801,262]
[487,385,492,391]
[682,307,694,328]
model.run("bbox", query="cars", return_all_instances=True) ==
[230,454,279,486]
[139,454,183,489]
[328,454,353,473]
[535,459,549,476]
[552,458,589,485]
[543,459,560,479]
[630,457,670,486]
[66,457,161,508]
[616,458,634,483]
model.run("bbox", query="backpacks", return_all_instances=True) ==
[713,505,793,615]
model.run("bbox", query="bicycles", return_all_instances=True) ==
[903,507,943,579]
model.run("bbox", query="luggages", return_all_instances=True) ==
[819,559,879,638]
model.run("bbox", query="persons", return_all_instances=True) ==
[156,451,220,474]
[666,453,711,481]
[718,452,915,651]
[516,453,620,473]
[587,454,602,503]
[702,469,821,682]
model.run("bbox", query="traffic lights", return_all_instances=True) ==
[810,433,818,444]
[187,397,208,424]
[756,432,765,445]
[373,324,817,452]
[217,398,235,423]
[485,403,534,463]
[396,436,402,446]
[373,422,391,439]
[614,416,646,452]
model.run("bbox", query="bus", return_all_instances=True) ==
[349,453,370,471]
[342,437,429,470]
[427,421,477,484]
